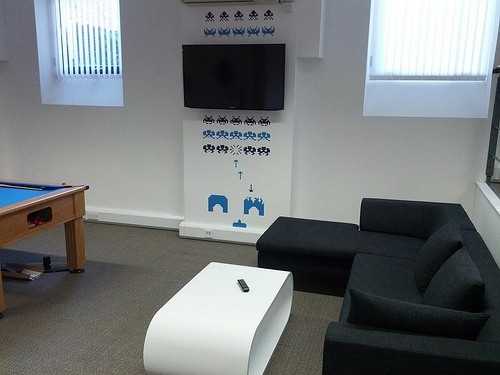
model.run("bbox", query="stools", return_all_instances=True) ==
[256,217,359,297]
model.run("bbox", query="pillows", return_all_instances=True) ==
[346,288,491,342]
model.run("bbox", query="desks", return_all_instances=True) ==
[0,180,89,319]
[143,262,293,375]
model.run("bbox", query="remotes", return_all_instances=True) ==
[238,279,249,292]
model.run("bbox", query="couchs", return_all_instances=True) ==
[322,198,500,375]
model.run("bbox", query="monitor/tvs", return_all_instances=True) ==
[182,43,286,111]
